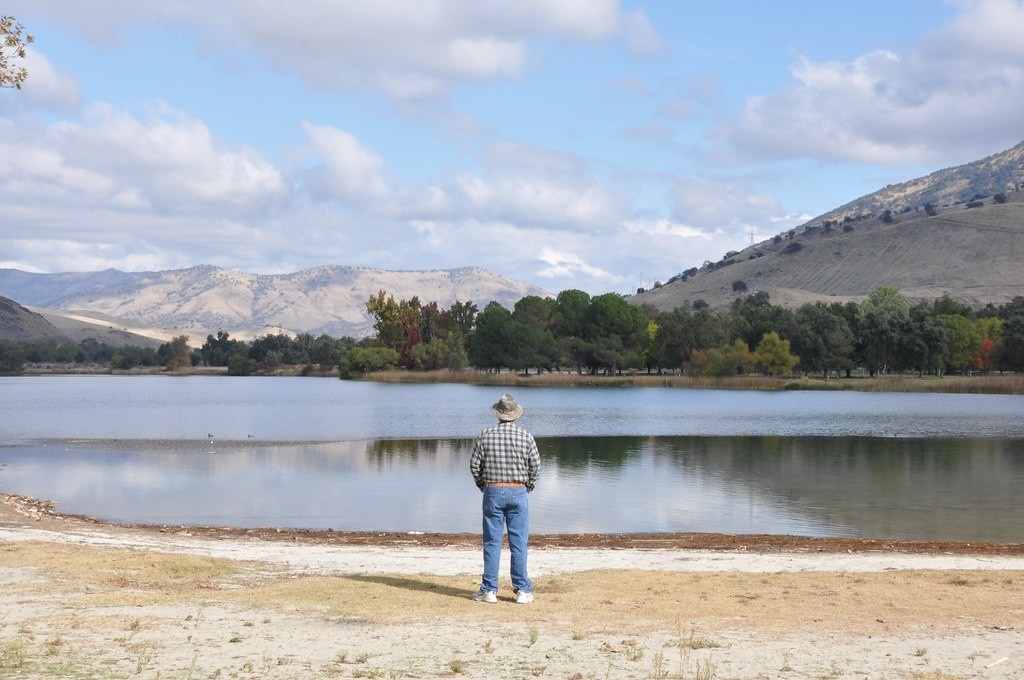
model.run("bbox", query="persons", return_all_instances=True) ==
[471,396,540,603]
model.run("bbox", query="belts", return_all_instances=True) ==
[483,482,526,488]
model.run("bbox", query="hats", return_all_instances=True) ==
[491,393,524,421]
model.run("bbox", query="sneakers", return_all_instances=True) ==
[516,590,534,604]
[472,589,498,603]
